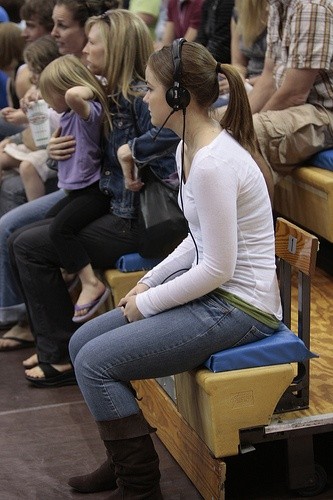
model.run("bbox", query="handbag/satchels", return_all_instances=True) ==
[133,170,188,260]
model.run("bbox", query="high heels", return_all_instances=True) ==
[21,353,40,369]
[23,363,78,386]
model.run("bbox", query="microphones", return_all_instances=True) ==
[153,109,176,142]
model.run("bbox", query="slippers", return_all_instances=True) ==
[0,324,39,350]
[72,287,110,322]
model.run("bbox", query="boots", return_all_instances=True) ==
[69,443,117,492]
[97,412,163,499]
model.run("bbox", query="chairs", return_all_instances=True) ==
[174,217,321,459]
[97,253,158,314]
[274,151,333,242]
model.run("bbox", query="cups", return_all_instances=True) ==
[27,100,49,146]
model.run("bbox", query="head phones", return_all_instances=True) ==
[166,38,191,109]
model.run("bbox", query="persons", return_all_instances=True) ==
[7,10,180,386]
[208,0,333,207]
[65,39,284,500]
[0,0,118,351]
[127,1,268,107]
